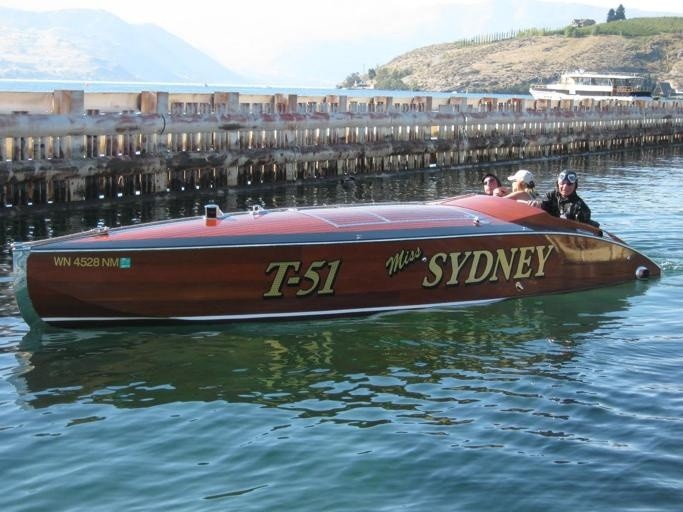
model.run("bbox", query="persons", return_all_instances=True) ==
[493,169,542,200]
[527,170,590,223]
[482,174,501,195]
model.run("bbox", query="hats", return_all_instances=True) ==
[506,170,535,188]
[556,170,578,184]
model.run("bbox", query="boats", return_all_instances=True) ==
[527,69,660,108]
[11,186,668,342]
